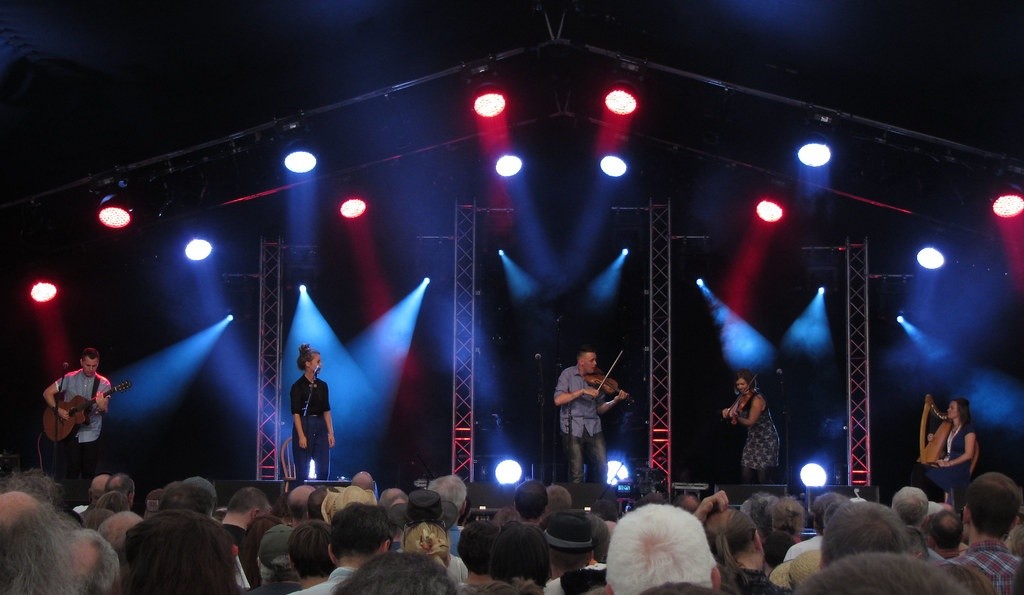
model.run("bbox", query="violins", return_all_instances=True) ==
[730,388,755,425]
[585,367,635,407]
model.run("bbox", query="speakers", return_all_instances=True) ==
[714,483,788,513]
[806,485,893,529]
[553,482,618,523]
[212,479,288,520]
[304,479,379,502]
[457,481,520,527]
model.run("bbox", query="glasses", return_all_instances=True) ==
[403,519,447,545]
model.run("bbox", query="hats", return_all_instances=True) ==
[259,524,295,572]
[387,489,458,529]
[182,477,216,517]
[321,486,377,524]
[544,511,601,554]
[145,490,162,515]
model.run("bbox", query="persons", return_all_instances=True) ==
[251,472,471,595]
[37,347,111,488]
[289,343,333,487]
[555,351,629,508]
[456,480,791,595]
[0,473,269,595]
[723,369,780,495]
[910,397,982,501]
[769,471,1024,594]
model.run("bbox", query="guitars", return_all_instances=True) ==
[43,379,133,445]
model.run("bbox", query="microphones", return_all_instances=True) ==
[63,362,69,368]
[535,354,541,359]
[314,366,320,374]
[776,368,784,375]
